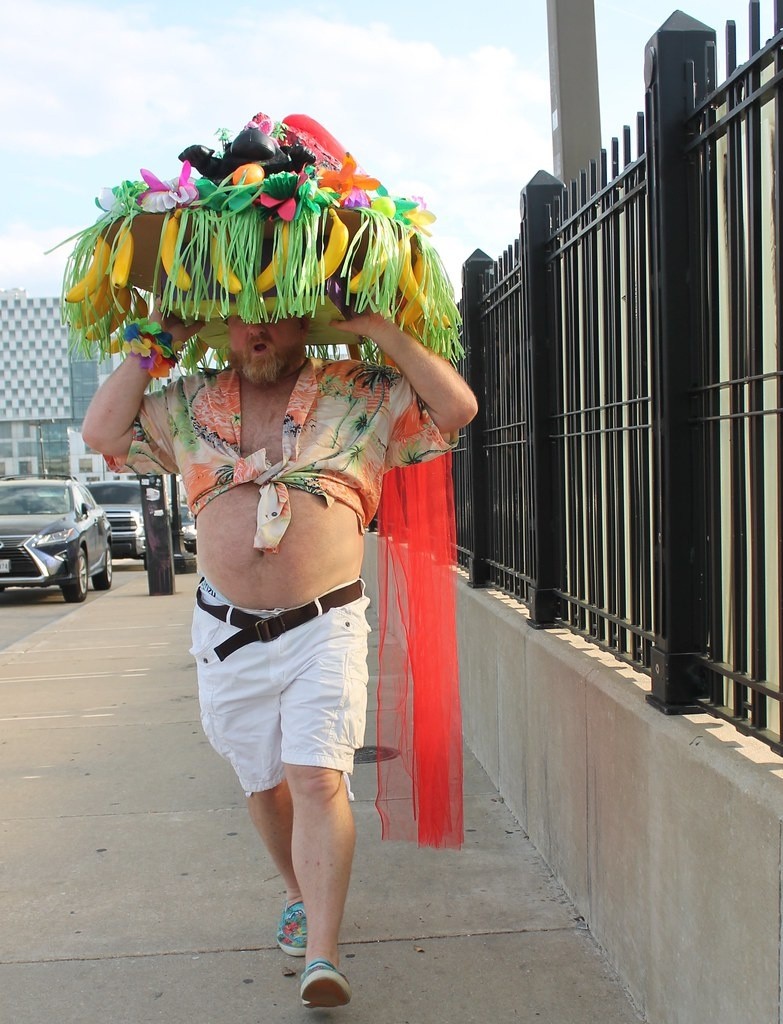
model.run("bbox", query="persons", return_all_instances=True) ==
[81,294,476,1010]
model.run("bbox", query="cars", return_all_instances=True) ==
[178,505,197,553]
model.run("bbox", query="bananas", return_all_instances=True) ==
[63,209,452,368]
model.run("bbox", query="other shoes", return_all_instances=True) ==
[300,957,352,1008]
[276,901,307,958]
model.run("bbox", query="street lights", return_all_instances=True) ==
[28,419,55,480]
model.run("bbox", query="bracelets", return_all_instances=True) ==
[121,318,187,381]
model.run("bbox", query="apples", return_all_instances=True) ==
[371,198,396,218]
[233,164,265,186]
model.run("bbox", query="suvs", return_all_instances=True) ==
[83,479,149,569]
[0,473,114,603]
